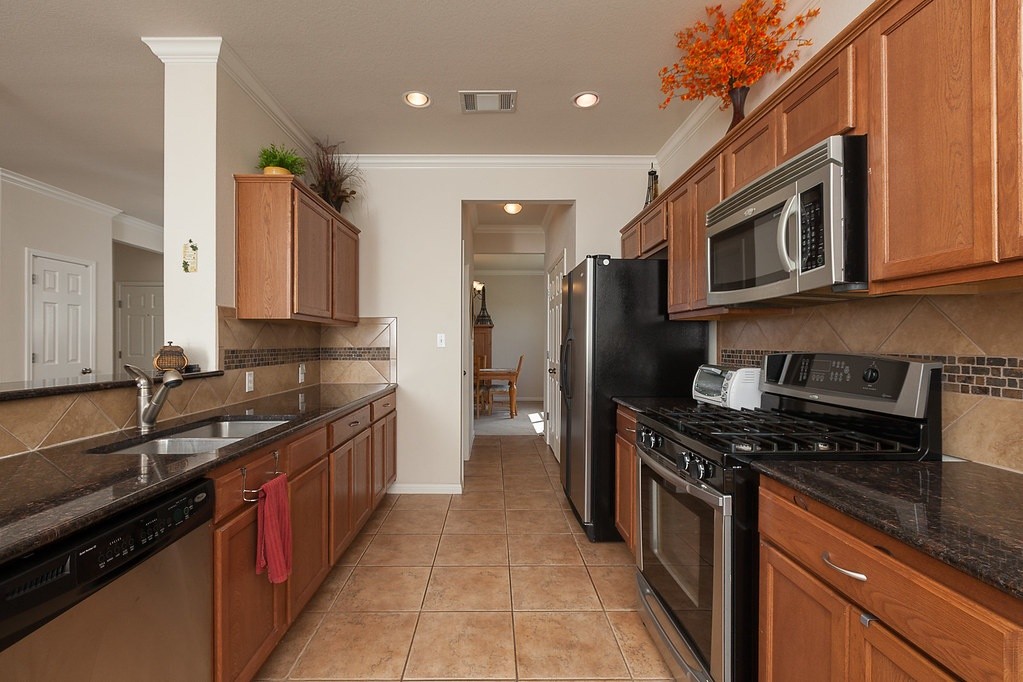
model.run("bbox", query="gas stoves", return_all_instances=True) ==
[636,350,943,495]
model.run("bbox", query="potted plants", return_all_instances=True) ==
[255,142,307,176]
[310,135,367,214]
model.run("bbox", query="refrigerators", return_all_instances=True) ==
[559,255,708,543]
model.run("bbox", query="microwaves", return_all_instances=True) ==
[706,135,868,304]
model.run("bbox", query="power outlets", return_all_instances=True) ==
[298,392,305,410]
[246,371,254,392]
[299,366,305,383]
[245,408,255,415]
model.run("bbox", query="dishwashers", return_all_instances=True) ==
[0,477,215,682]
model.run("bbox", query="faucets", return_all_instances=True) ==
[122,364,184,431]
[136,454,168,487]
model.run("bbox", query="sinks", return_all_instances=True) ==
[108,438,245,456]
[164,420,289,438]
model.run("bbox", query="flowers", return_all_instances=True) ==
[659,0,818,108]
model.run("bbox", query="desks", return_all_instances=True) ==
[473,369,518,420]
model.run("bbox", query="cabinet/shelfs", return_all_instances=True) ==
[233,173,362,327]
[329,397,372,568]
[868,0,1023,295]
[212,420,329,681]
[721,18,858,199]
[613,405,636,559]
[756,473,1022,682]
[372,390,399,512]
[618,189,667,259]
[667,143,725,321]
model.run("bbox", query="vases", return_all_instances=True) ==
[726,87,749,132]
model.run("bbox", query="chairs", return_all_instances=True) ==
[473,356,487,420]
[488,355,524,416]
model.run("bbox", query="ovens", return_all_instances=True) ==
[636,441,759,682]
[693,363,762,410]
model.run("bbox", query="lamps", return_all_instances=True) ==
[503,204,522,215]
[472,280,486,300]
